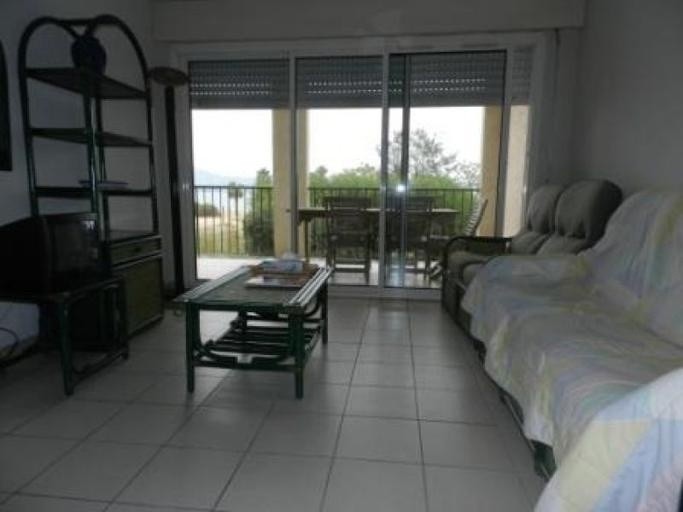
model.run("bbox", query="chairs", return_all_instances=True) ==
[298,188,488,287]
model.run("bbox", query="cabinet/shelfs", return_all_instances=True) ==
[17,12,163,338]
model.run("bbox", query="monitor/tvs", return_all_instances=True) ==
[0,212,101,305]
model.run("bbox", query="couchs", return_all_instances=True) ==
[445,176,624,352]
[455,188,681,512]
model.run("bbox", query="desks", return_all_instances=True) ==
[172,256,333,398]
[0,275,133,395]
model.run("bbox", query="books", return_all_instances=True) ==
[245,274,308,289]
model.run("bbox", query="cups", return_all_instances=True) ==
[78,220,96,240]
